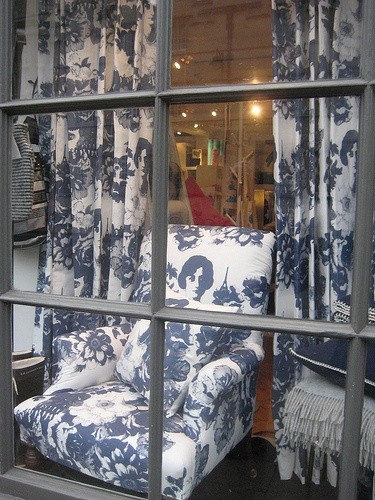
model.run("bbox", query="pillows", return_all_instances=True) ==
[287,328,375,396]
[112,280,244,415]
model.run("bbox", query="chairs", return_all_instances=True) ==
[278,287,374,500]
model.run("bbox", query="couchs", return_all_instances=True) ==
[12,224,274,500]
